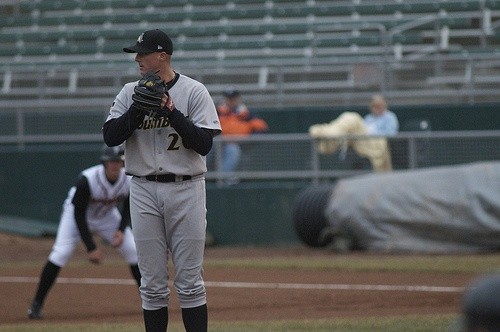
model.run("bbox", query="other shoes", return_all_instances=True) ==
[27,309,42,319]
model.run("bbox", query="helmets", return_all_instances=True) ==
[101,144,125,166]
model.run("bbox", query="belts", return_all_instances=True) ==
[134,173,191,183]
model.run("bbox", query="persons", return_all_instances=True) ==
[216,87,269,173]
[28,143,142,320]
[102,28,223,332]
[363,95,401,171]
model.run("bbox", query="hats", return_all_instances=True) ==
[223,86,238,96]
[122,29,173,55]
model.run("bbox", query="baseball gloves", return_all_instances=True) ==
[132,67,173,119]
